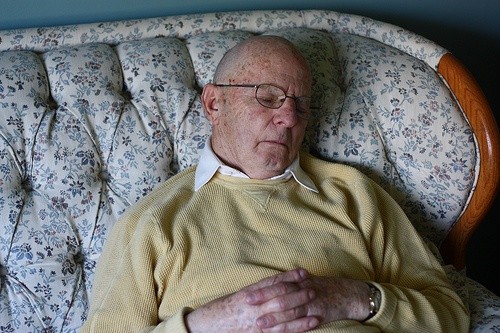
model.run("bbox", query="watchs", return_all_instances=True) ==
[366,281,381,321]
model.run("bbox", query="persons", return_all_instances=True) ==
[74,34,474,333]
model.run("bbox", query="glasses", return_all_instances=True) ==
[212,79,314,119]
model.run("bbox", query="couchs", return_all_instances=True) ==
[0,8,500,333]
